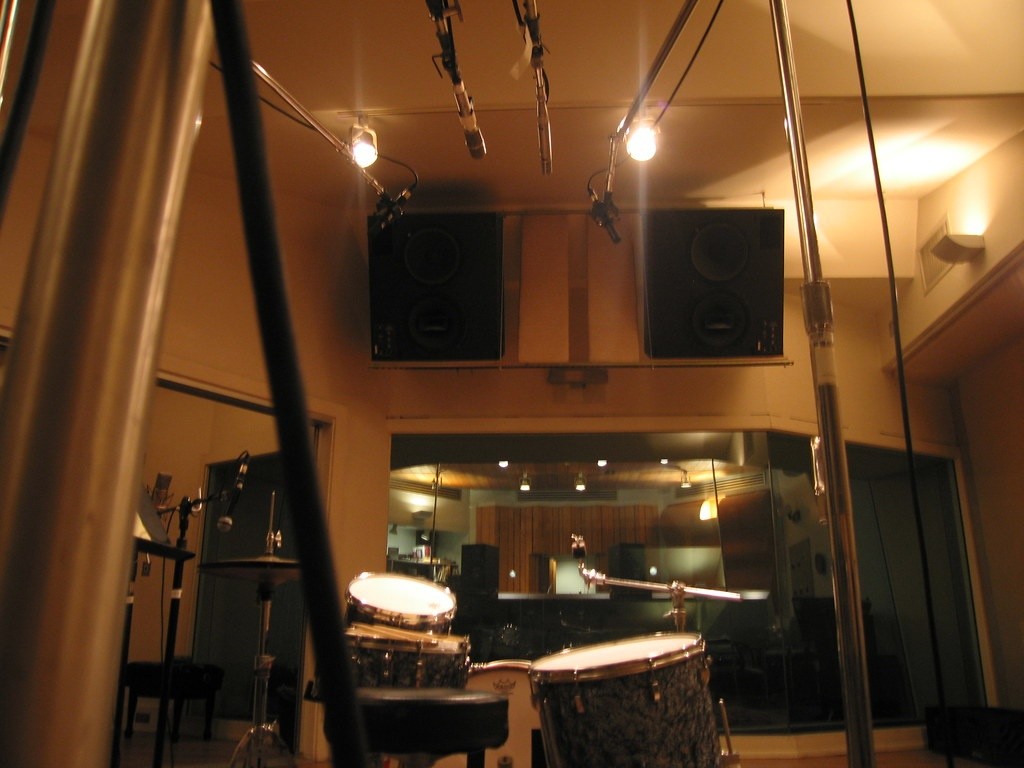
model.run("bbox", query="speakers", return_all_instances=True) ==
[460,544,499,599]
[647,208,785,358]
[366,210,505,362]
[609,543,652,602]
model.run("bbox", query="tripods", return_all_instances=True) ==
[228,570,294,768]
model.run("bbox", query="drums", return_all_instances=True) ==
[347,573,459,635]
[389,561,458,597]
[529,630,739,767]
[343,621,472,690]
[436,661,545,768]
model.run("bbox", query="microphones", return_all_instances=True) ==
[215,455,250,533]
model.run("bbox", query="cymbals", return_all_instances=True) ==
[198,554,301,579]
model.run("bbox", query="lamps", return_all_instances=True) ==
[421,530,431,541]
[574,463,587,491]
[929,233,986,264]
[431,471,441,490]
[518,464,531,491]
[388,523,399,536]
[680,469,691,488]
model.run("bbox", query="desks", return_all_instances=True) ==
[351,687,509,768]
[123,665,218,743]
